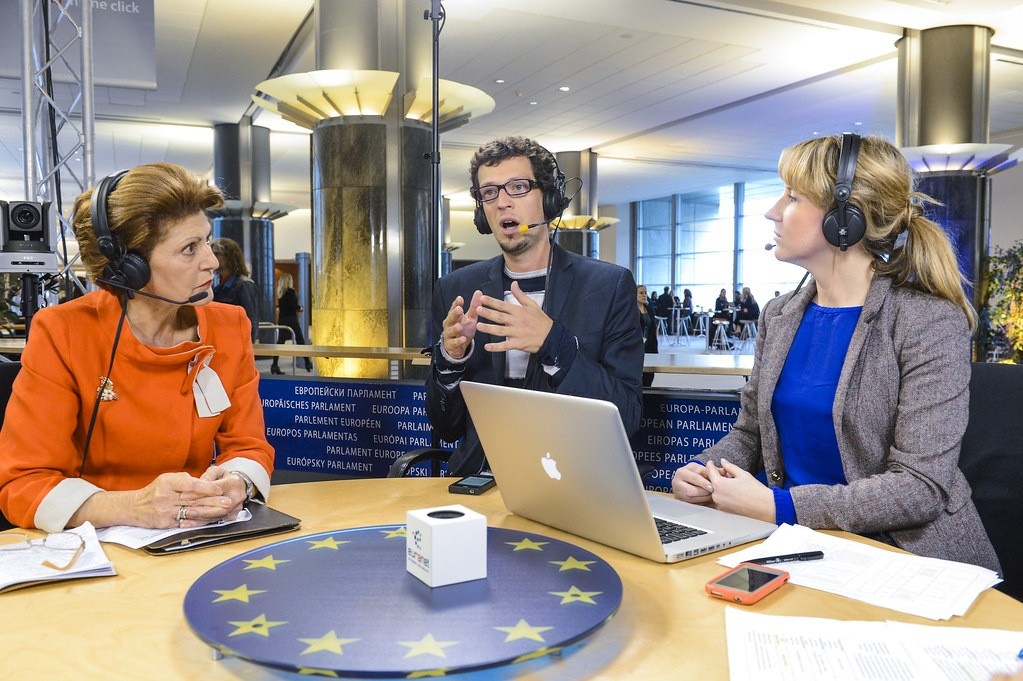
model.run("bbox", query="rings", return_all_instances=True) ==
[176,509,183,521]
[183,509,186,518]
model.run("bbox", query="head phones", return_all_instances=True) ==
[473,146,569,234]
[91,170,152,291]
[822,132,867,252]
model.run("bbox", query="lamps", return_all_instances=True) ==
[442,241,466,254]
[548,216,621,232]
[206,199,299,222]
[251,67,496,133]
[900,143,1023,179]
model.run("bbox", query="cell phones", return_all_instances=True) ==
[706,561,789,605]
[448,474,495,495]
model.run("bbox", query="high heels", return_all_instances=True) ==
[271,364,285,375]
[306,362,312,372]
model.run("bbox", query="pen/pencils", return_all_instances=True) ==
[740,551,824,564]
[1007,649,1023,674]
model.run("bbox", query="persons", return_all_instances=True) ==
[668,291,677,304]
[271,273,312,375]
[0,163,275,529]
[733,287,760,340]
[675,289,693,319]
[637,285,658,387]
[670,134,1005,591]
[655,287,673,336]
[425,139,644,478]
[715,289,735,338]
[9,285,22,316]
[209,238,258,344]
[650,291,657,316]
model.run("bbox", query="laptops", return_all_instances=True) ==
[459,380,780,564]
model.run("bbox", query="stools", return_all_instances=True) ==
[655,316,758,356]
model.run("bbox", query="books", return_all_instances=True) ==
[0,522,117,593]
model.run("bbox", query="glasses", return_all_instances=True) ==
[473,178,541,202]
[0,532,85,571]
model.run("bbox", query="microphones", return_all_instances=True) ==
[517,206,564,233]
[98,278,209,305]
[764,243,776,250]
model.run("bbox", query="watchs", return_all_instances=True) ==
[231,472,255,505]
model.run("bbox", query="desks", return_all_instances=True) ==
[0,479,1023,680]
[696,310,722,355]
[666,307,691,348]
